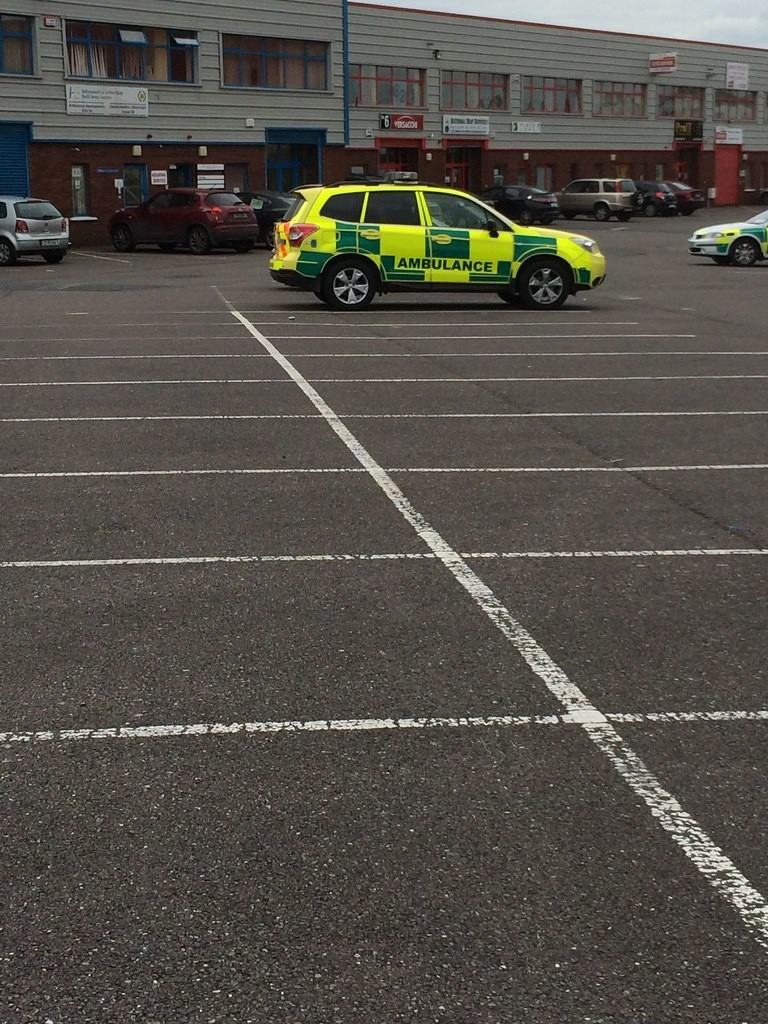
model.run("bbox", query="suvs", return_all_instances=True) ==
[269,179,606,310]
[553,178,645,223]
[634,181,678,217]
[108,188,259,255]
[686,209,768,267]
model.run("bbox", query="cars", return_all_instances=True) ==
[0,195,70,267]
[475,184,560,226]
[669,180,706,217]
[232,191,298,250]
[287,183,325,195]
[758,188,768,206]
